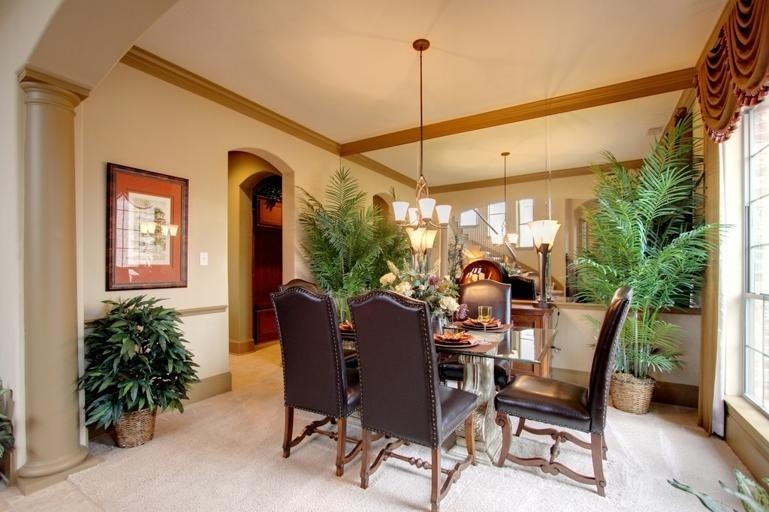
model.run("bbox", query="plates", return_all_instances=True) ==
[337,321,355,333]
[463,319,502,330]
[433,333,479,349]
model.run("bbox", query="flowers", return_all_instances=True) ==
[379,247,472,322]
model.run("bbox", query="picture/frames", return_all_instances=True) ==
[106,161,189,292]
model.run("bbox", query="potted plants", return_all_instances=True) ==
[565,112,736,416]
[73,296,201,449]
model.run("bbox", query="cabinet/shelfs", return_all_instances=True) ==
[511,304,557,379]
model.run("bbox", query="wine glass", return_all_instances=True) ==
[477,306,492,344]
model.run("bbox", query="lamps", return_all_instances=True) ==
[138,217,179,240]
